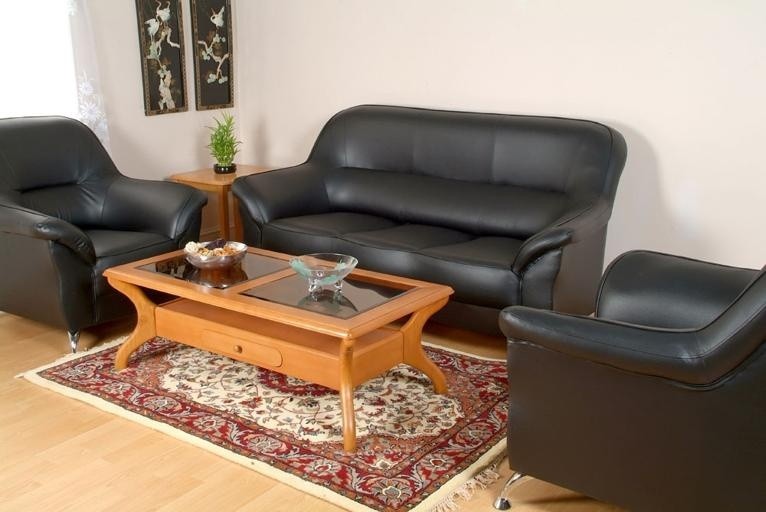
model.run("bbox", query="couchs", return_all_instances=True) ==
[231,104,626,316]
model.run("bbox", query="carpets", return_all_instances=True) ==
[15,334,508,512]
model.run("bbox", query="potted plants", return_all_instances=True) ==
[205,109,241,173]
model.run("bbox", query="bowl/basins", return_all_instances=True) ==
[289,251,358,291]
[183,239,248,270]
[294,291,358,318]
[187,270,249,288]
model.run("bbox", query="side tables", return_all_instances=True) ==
[169,166,275,244]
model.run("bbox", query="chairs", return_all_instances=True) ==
[493,250,765,511]
[0,116,209,353]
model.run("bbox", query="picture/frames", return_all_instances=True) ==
[136,0,234,115]
